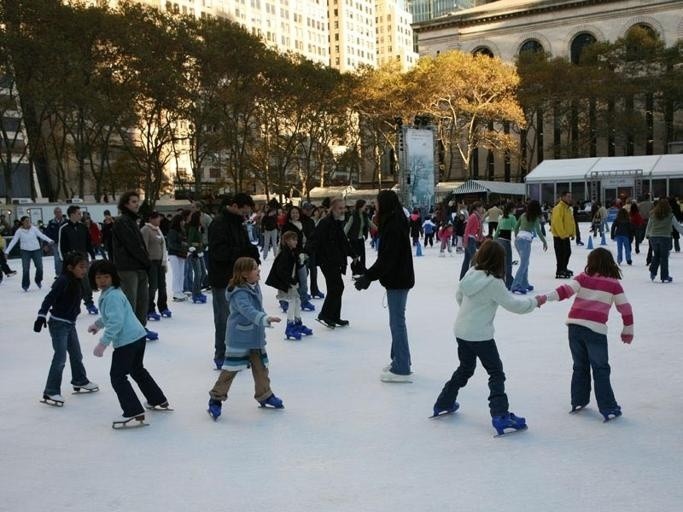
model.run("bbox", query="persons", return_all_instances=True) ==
[82,260,173,423]
[428,236,541,434]
[1,189,683,338]
[537,245,638,420]
[206,256,287,416]
[353,191,419,382]
[33,250,98,403]
[210,193,262,368]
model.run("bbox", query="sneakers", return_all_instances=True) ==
[523,284,533,291]
[208,396,222,417]
[311,290,323,297]
[492,413,524,430]
[650,272,655,278]
[215,359,224,369]
[660,276,671,281]
[144,327,158,340]
[301,302,314,309]
[192,294,205,303]
[381,364,412,372]
[646,257,652,262]
[86,303,97,313]
[285,326,301,339]
[575,240,584,245]
[570,397,590,406]
[160,308,171,317]
[147,312,159,320]
[380,370,412,382]
[433,401,459,412]
[71,381,98,391]
[43,391,64,403]
[511,285,526,293]
[627,260,632,264]
[144,399,168,408]
[173,293,185,300]
[257,393,283,406]
[112,410,144,423]
[599,403,621,416]
[279,300,288,311]
[297,325,312,334]
[335,318,348,326]
[318,311,336,327]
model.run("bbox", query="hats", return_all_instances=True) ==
[302,201,309,210]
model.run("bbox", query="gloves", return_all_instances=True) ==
[87,322,99,335]
[621,327,633,343]
[354,274,370,291]
[92,343,105,357]
[264,316,281,328]
[33,316,46,332]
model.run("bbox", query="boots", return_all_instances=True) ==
[556,263,573,276]
[1,264,15,276]
